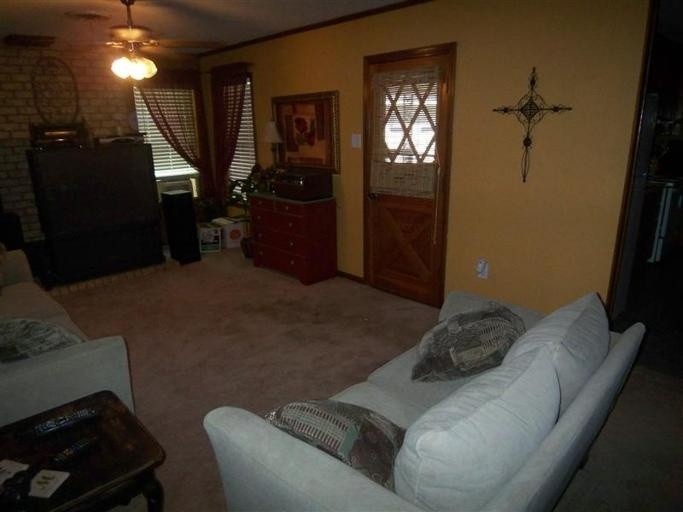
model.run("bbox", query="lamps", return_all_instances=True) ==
[111,50,159,82]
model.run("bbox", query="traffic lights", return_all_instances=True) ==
[0,317,86,366]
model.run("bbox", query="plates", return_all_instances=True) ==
[245,187,339,287]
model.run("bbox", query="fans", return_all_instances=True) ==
[70,0,227,60]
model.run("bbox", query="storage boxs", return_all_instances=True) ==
[195,213,250,254]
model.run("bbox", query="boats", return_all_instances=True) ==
[162,188,202,264]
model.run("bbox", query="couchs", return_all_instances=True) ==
[0,249,138,427]
[203,289,645,512]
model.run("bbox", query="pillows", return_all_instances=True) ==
[265,398,409,484]
[412,305,525,381]
[0,317,86,366]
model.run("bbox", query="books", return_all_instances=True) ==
[195,213,250,254]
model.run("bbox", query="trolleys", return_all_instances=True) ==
[70,0,227,60]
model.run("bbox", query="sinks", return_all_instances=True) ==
[0,249,138,427]
[203,289,645,512]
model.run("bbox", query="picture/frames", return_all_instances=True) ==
[268,89,343,174]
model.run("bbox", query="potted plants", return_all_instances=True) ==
[268,89,343,174]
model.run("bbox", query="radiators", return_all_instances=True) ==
[33,407,98,435]
[54,434,93,463]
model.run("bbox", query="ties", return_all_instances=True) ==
[24,142,159,237]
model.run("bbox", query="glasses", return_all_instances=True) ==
[111,50,159,82]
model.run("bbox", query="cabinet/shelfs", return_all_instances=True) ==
[245,187,339,287]
